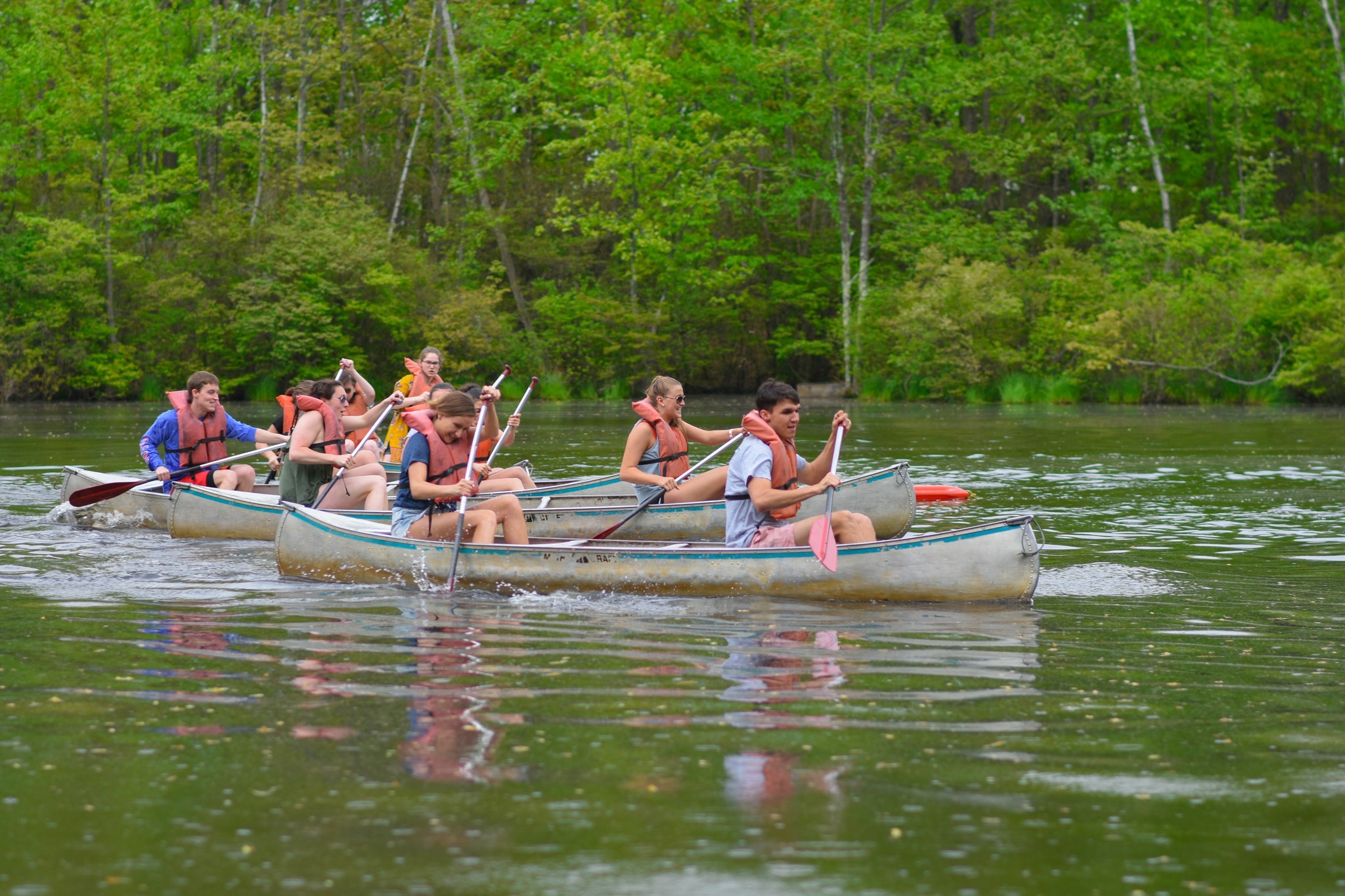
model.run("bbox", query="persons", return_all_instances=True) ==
[726,377,876,547]
[619,376,747,505]
[256,347,537,544]
[139,371,290,490]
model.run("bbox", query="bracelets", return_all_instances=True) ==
[729,429,734,440]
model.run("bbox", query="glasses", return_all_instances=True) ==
[331,397,348,406]
[345,386,352,394]
[655,396,686,404]
[422,360,441,367]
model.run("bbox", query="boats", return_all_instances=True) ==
[456,459,916,544]
[165,471,641,541]
[53,452,402,528]
[913,485,970,501]
[273,501,1047,605]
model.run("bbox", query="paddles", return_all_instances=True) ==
[311,396,399,511]
[265,409,305,484]
[809,420,845,573]
[446,392,488,590]
[477,374,538,488]
[68,440,288,507]
[336,363,346,381]
[490,366,510,392]
[591,429,750,541]
